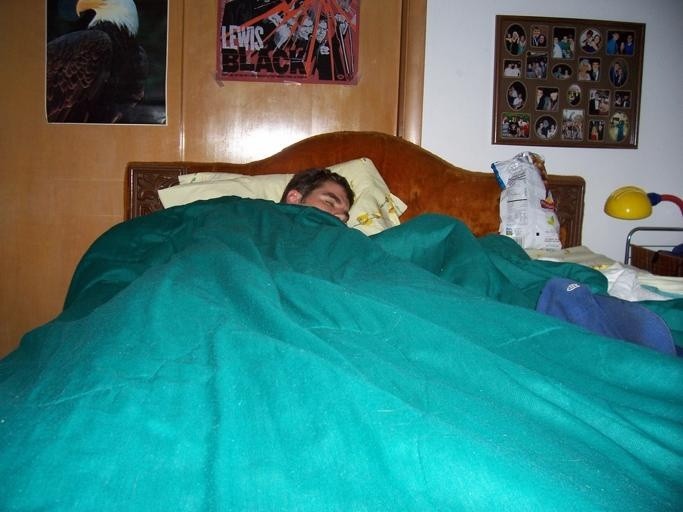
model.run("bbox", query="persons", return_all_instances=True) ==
[298,16,314,58]
[611,62,619,83]
[612,117,625,142]
[623,35,633,55]
[581,30,593,49]
[615,93,631,108]
[616,68,625,86]
[562,114,583,141]
[588,61,599,81]
[505,34,512,50]
[607,32,619,55]
[520,36,526,52]
[537,89,545,110]
[525,54,548,80]
[313,19,329,56]
[538,34,546,46]
[545,89,557,111]
[588,120,604,142]
[578,59,591,81]
[511,32,519,54]
[505,64,512,76]
[536,122,546,138]
[547,124,555,138]
[589,89,609,118]
[514,63,520,76]
[553,65,571,80]
[553,33,574,59]
[587,35,600,52]
[509,86,524,110]
[280,167,355,225]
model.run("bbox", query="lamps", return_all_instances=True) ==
[604,186,683,220]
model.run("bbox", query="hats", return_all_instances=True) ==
[536,276,676,357]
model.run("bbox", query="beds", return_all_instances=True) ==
[0,130,683,511]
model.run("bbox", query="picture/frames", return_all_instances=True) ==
[491,14,646,149]
